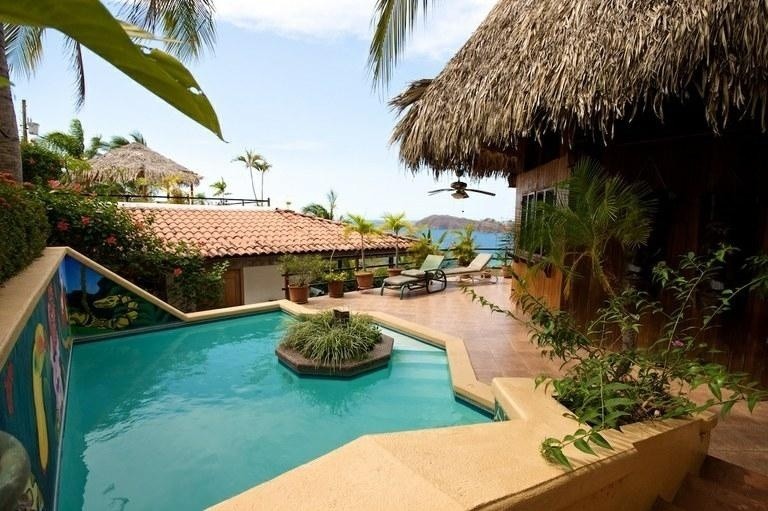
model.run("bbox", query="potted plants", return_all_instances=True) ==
[277,254,349,304]
[496,252,512,278]
[447,222,480,279]
[341,207,417,290]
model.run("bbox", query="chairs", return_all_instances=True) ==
[381,252,493,300]
